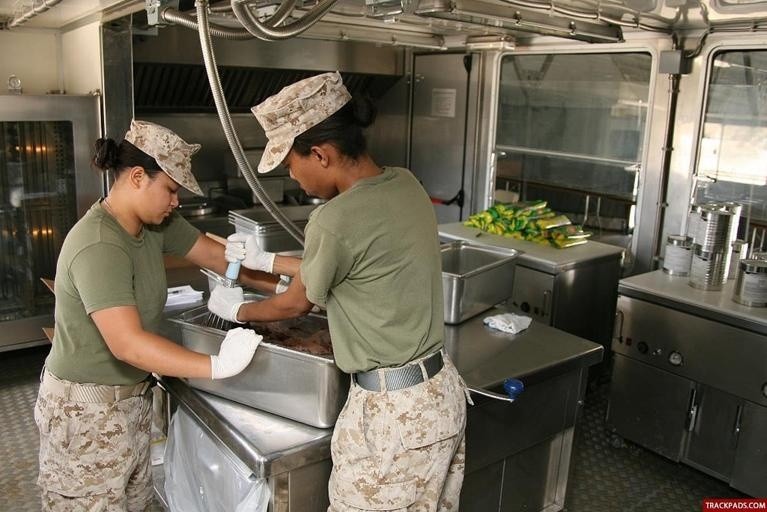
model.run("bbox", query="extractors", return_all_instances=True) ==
[135,34,398,114]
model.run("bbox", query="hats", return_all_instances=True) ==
[249,69,353,175]
[124,118,205,197]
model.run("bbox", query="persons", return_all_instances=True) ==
[34,116,315,512]
[207,72,474,512]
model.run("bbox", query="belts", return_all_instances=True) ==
[350,352,444,393]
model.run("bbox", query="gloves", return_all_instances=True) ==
[275,279,326,313]
[206,284,255,325]
[224,232,275,275]
[210,325,264,381]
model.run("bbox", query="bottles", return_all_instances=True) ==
[661,202,767,309]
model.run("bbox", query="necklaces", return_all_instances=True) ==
[103,199,115,214]
